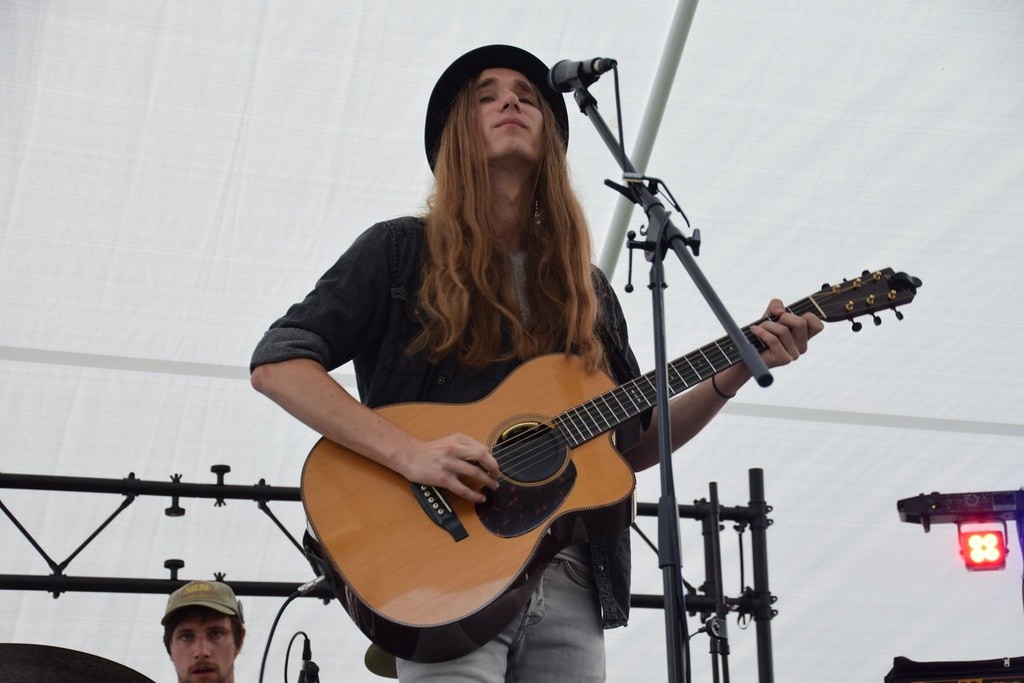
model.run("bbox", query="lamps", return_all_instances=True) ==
[957,516,1009,569]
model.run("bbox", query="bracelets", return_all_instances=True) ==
[712,376,737,399]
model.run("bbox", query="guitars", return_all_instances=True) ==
[300,266,924,666]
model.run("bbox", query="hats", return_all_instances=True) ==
[161,581,245,624]
[425,44,568,177]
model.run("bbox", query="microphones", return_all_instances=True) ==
[548,58,618,92]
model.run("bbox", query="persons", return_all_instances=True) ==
[162,580,246,683]
[252,35,825,683]
